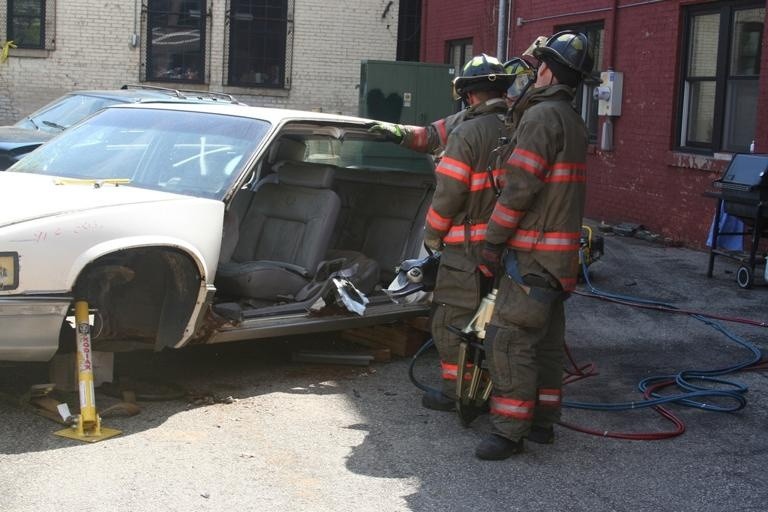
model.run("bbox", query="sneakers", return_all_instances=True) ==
[474,425,554,460]
[422,390,457,412]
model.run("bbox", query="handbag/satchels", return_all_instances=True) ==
[489,273,563,330]
[432,252,482,311]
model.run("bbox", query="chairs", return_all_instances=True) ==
[215,136,438,303]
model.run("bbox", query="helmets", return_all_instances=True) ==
[452,52,535,102]
[531,29,603,85]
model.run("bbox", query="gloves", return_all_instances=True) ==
[424,237,443,251]
[360,120,403,145]
